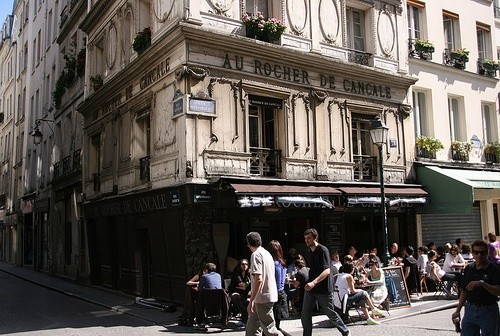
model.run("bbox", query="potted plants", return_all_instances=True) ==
[131,25,152,56]
[447,48,470,69]
[482,59,500,71]
[90,73,104,92]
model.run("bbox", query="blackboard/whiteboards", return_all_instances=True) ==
[380,266,411,309]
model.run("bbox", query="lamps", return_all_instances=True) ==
[31,119,54,146]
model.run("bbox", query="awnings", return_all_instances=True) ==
[230,183,343,196]
[340,187,428,196]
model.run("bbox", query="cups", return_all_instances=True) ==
[453,319,461,333]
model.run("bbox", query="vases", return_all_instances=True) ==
[417,147,437,159]
[415,44,434,53]
[452,149,469,161]
[244,21,282,45]
[484,147,493,153]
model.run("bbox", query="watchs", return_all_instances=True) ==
[480,280,484,288]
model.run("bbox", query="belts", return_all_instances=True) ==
[470,303,487,307]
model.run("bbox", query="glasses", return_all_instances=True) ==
[472,250,486,255]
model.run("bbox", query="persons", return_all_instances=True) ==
[452,240,500,336]
[186,263,222,324]
[246,232,290,336]
[384,238,473,299]
[331,246,388,324]
[288,254,309,314]
[301,229,350,336]
[488,234,500,264]
[231,259,252,326]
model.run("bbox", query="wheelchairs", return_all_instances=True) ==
[196,288,231,326]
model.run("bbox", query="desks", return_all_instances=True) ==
[356,282,380,323]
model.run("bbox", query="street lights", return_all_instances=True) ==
[368,115,392,267]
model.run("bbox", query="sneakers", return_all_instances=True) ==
[367,319,378,325]
[372,309,382,315]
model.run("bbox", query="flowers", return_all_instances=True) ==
[415,39,434,48]
[485,140,500,159]
[415,135,445,153]
[241,10,287,41]
[451,140,474,157]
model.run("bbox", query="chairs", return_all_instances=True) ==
[430,269,455,300]
[419,271,428,294]
[195,288,230,331]
[332,285,364,326]
